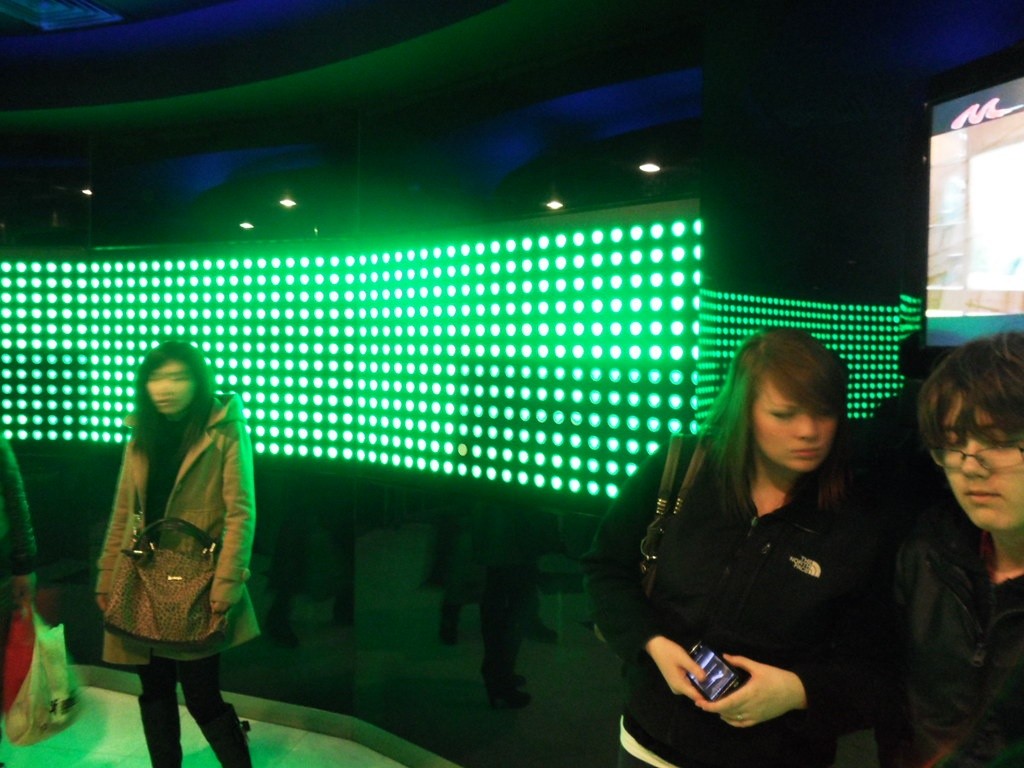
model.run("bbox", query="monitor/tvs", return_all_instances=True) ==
[901,44,1024,377]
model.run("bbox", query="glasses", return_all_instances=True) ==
[927,442,1024,472]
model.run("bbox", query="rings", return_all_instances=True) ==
[737,711,743,722]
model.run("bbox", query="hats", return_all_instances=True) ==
[897,330,945,376]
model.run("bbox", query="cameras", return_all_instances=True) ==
[681,638,743,703]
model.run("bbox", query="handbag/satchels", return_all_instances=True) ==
[5,604,88,746]
[588,436,711,643]
[0,587,59,714]
[102,516,230,653]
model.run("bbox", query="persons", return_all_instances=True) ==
[857,329,1024,768]
[271,470,561,710]
[95,339,261,768]
[0,429,36,706]
[580,328,900,768]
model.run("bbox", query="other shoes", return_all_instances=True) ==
[332,604,355,628]
[261,613,299,649]
[518,620,558,644]
[439,599,460,647]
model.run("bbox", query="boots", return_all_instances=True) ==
[195,702,252,768]
[137,689,183,768]
[479,600,532,709]
